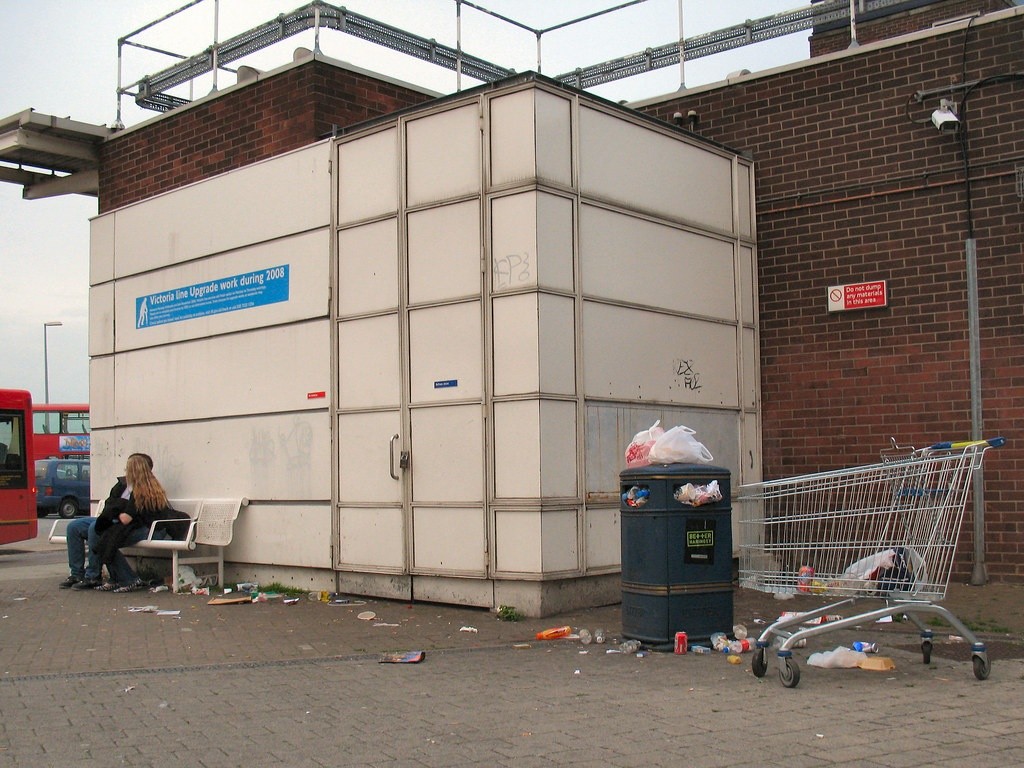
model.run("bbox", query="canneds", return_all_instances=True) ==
[674,630,687,655]
[852,641,879,653]
[798,566,814,593]
[156,586,168,592]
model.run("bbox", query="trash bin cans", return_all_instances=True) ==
[620,463,736,653]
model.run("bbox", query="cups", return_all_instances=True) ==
[673,483,696,501]
[734,624,747,639]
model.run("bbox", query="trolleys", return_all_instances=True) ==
[737,435,1007,688]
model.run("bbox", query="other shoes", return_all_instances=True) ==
[59,575,85,589]
[94,581,122,591]
[71,576,104,591]
[113,578,142,593]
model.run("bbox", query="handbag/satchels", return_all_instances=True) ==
[625,419,713,470]
[844,546,927,592]
[167,509,190,540]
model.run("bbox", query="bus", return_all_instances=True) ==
[0,387,38,546]
[31,402,90,461]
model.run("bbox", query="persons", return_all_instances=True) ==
[57,455,170,593]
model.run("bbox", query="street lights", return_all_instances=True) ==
[44,322,63,432]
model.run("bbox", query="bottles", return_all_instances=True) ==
[723,637,756,653]
[623,489,649,500]
[236,583,259,592]
[537,626,571,640]
[579,628,592,644]
[620,639,641,655]
[308,592,336,602]
[593,626,605,644]
[775,636,806,648]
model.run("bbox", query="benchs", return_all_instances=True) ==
[48,496,250,593]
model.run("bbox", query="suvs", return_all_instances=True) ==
[35,458,90,519]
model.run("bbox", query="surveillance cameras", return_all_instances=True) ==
[932,110,961,133]
